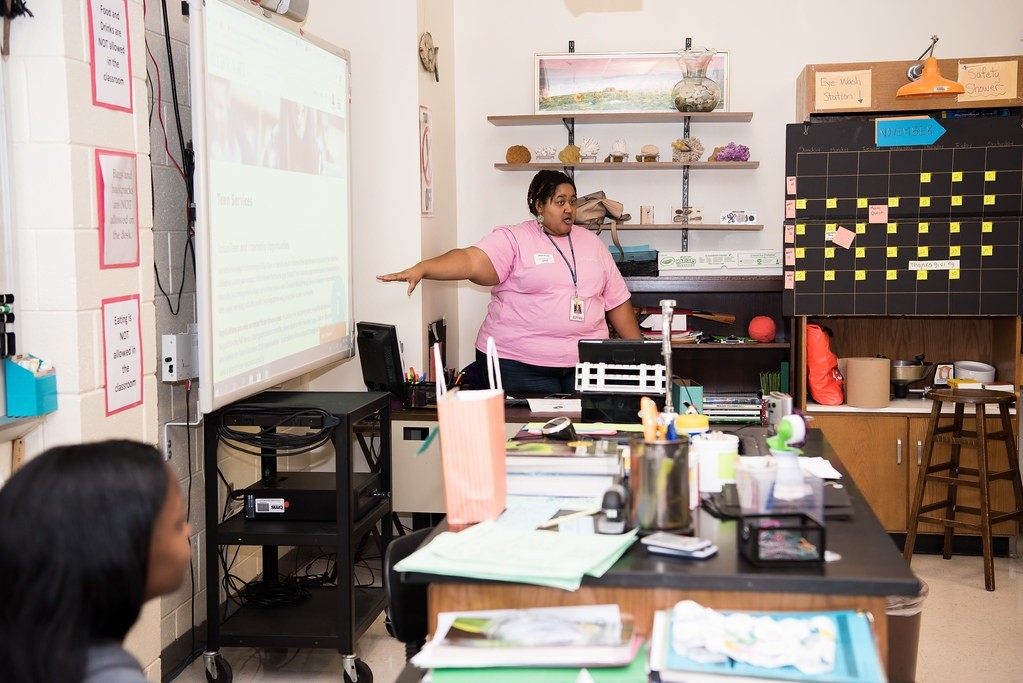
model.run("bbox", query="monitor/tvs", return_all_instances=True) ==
[355,321,407,403]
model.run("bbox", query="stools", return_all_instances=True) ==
[903,388,1023,592]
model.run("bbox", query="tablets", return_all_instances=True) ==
[577,339,669,423]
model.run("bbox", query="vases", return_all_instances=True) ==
[670,47,721,111]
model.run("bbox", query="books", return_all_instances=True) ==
[703,393,762,426]
[508,441,625,510]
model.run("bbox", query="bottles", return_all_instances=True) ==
[671,47,721,112]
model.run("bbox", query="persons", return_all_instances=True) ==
[0,439,193,683]
[377,169,643,399]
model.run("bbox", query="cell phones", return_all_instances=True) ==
[641,532,719,557]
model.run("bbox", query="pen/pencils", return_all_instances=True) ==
[443,367,465,390]
[404,366,427,406]
[633,395,691,536]
[637,396,657,441]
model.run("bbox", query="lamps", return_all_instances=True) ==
[896,34,966,98]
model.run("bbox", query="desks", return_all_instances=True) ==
[353,399,927,683]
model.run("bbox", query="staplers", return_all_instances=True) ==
[597,483,632,535]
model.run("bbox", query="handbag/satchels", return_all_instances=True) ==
[806,325,845,406]
[573,189,632,264]
[433,337,506,528]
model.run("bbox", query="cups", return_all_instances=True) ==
[629,436,690,529]
[733,466,777,511]
[693,435,739,493]
[420,381,437,405]
[405,383,419,407]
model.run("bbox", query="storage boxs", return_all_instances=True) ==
[796,55,1023,121]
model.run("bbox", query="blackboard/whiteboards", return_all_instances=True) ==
[189,1,357,413]
[783,106,1023,317]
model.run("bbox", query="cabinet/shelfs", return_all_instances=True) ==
[204,390,393,683]
[800,412,1020,538]
[488,111,794,425]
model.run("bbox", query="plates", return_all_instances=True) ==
[954,360,995,383]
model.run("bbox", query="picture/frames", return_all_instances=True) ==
[534,48,730,115]
